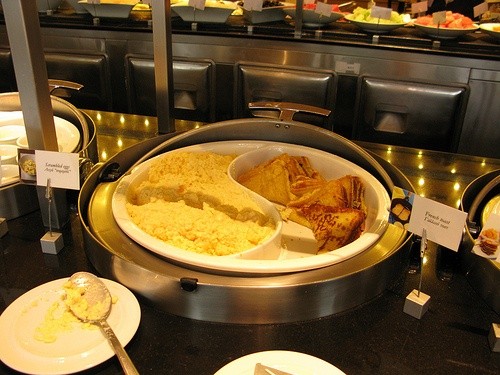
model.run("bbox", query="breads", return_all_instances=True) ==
[236,154,367,256]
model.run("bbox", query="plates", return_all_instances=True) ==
[111,140,392,275]
[0,110,80,191]
[481,196,500,261]
[78,0,140,19]
[480,22,500,38]
[170,1,236,23]
[341,12,406,35]
[0,274,142,374]
[210,350,347,375]
[282,5,344,27]
[412,18,479,39]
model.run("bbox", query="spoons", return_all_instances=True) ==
[65,271,142,375]
